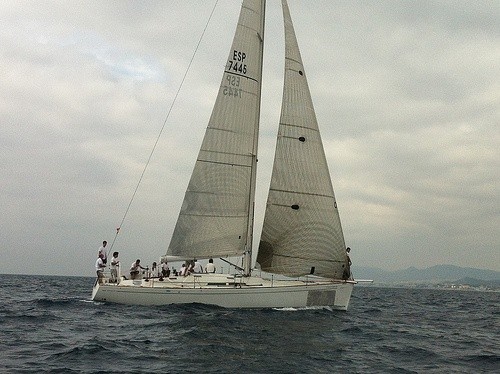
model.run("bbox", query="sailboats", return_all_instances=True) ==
[91,0,360,311]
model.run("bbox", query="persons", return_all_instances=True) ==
[95,254,106,278]
[346,247,352,265]
[205,259,216,273]
[110,251,120,279]
[99,241,108,277]
[130,259,147,280]
[151,262,169,277]
[181,259,203,276]
